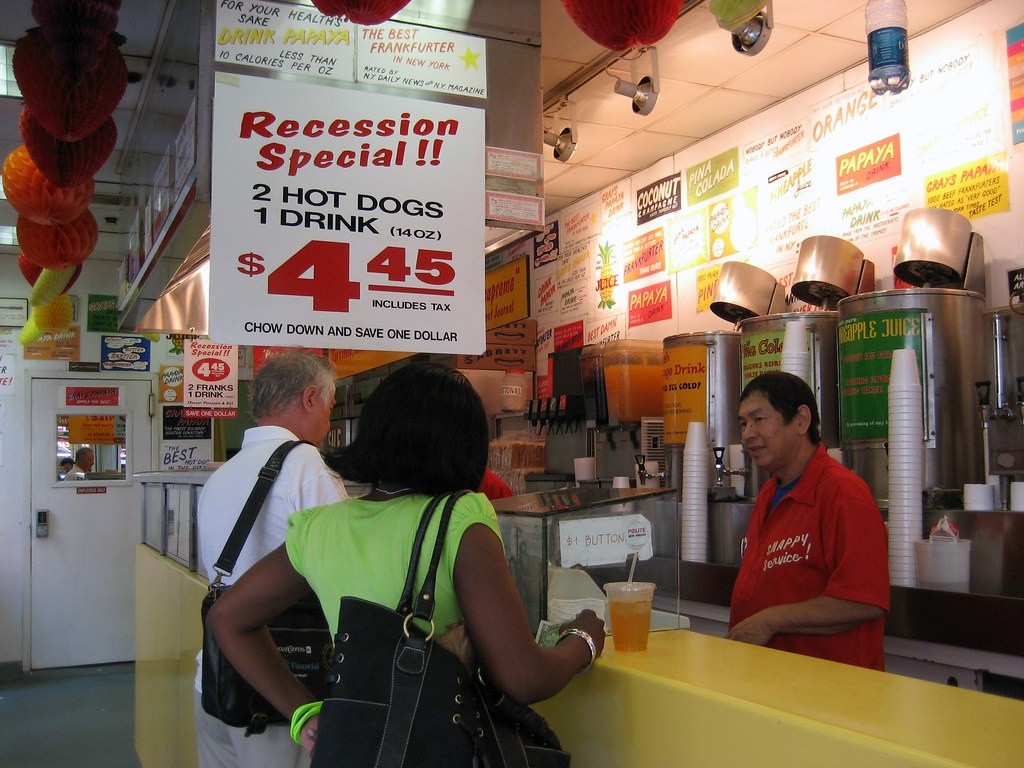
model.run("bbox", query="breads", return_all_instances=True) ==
[485,442,545,471]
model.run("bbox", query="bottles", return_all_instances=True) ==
[865,1,910,96]
[500,370,527,412]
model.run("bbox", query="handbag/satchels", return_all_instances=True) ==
[201,440,333,737]
[310,489,571,768]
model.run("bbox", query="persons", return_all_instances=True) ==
[63,447,95,481]
[205,362,606,756]
[723,371,889,670]
[194,351,347,768]
[59,458,75,474]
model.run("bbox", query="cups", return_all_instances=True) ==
[887,349,922,588]
[681,421,708,563]
[780,321,809,389]
[605,582,658,652]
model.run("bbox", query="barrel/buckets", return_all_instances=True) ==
[915,539,971,583]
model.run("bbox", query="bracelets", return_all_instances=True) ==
[556,629,595,673]
[289,701,322,744]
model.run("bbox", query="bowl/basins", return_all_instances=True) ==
[574,458,596,487]
[635,462,659,488]
[613,477,629,488]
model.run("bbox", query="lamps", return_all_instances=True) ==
[731,0,774,57]
[544,100,578,163]
[606,46,660,116]
